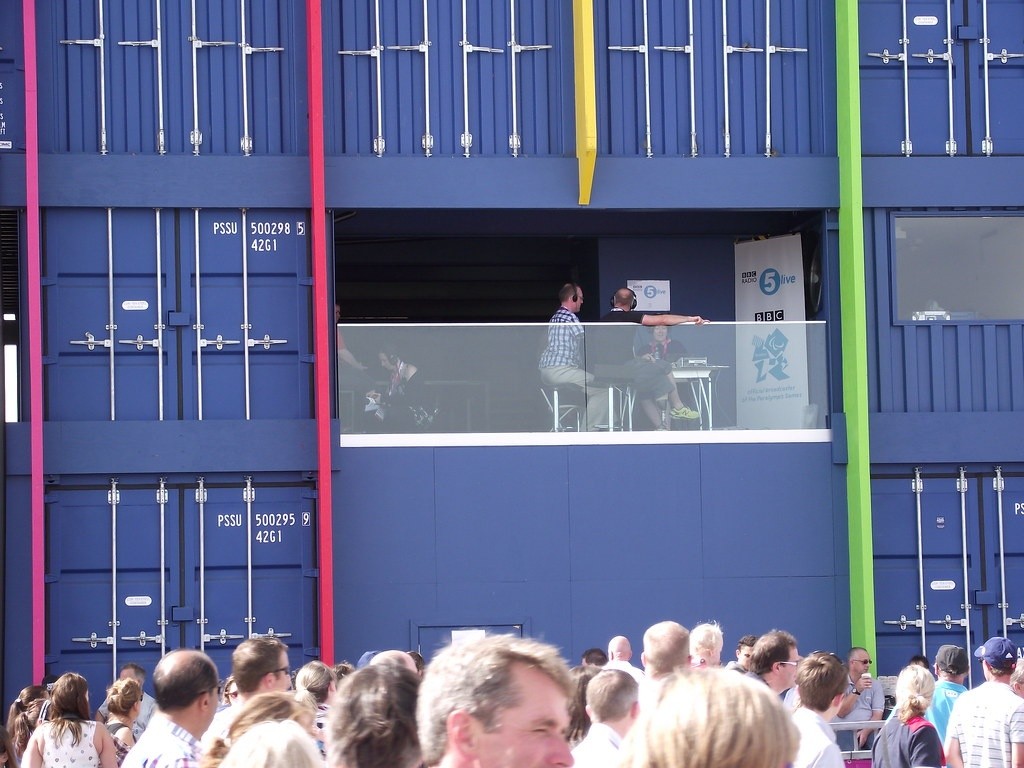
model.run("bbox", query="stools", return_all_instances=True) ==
[537,381,634,432]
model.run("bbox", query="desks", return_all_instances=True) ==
[377,379,492,433]
[660,366,732,430]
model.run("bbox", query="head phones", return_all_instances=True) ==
[571,282,578,302]
[610,288,637,309]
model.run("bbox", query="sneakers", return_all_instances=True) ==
[669,405,699,420]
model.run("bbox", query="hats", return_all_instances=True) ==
[358,650,380,670]
[974,637,1018,668]
[936,645,968,674]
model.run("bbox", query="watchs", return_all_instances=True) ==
[852,688,861,695]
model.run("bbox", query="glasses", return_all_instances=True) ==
[852,659,873,664]
[577,295,583,301]
[224,691,238,698]
[268,667,291,675]
[812,651,843,667]
[137,695,144,703]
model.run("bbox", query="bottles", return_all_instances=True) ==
[655,347,661,361]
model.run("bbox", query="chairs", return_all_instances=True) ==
[338,388,354,433]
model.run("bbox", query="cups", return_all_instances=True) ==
[862,673,872,689]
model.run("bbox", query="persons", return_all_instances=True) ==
[538,284,609,432]
[332,301,426,435]
[593,288,710,430]
[0,619,1024,768]
